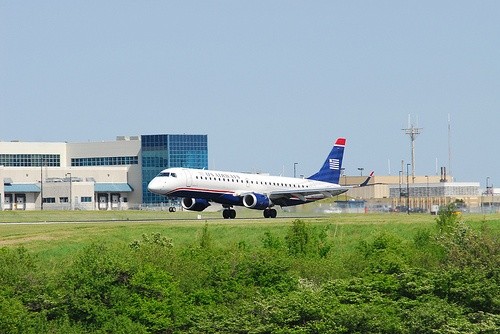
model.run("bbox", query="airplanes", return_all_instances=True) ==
[147,137,375,220]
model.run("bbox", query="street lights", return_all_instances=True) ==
[358,168,364,175]
[293,162,299,178]
[65,172,72,212]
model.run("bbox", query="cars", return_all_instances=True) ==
[389,205,423,213]
